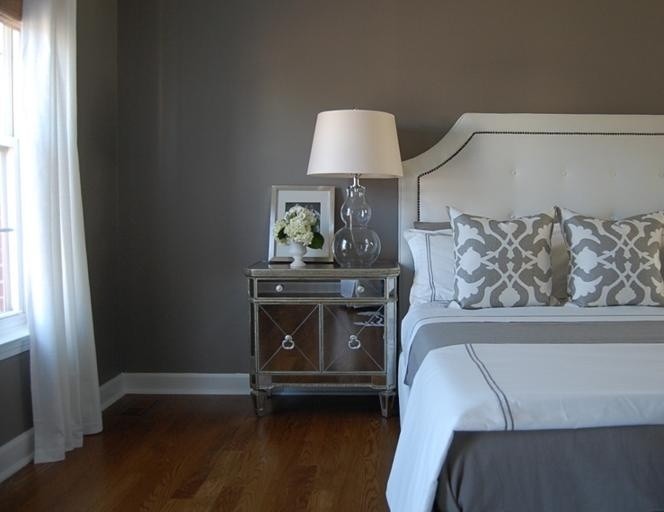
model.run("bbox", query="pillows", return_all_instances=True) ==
[446,205,559,309]
[557,207,664,307]
[404,229,455,303]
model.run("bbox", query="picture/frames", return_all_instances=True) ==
[268,184,336,264]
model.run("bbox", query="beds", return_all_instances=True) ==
[397,113,664,509]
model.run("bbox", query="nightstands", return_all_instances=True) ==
[243,260,401,418]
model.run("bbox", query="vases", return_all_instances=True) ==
[289,243,307,267]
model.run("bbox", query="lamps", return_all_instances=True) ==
[306,109,403,267]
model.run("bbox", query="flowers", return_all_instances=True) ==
[273,206,321,245]
